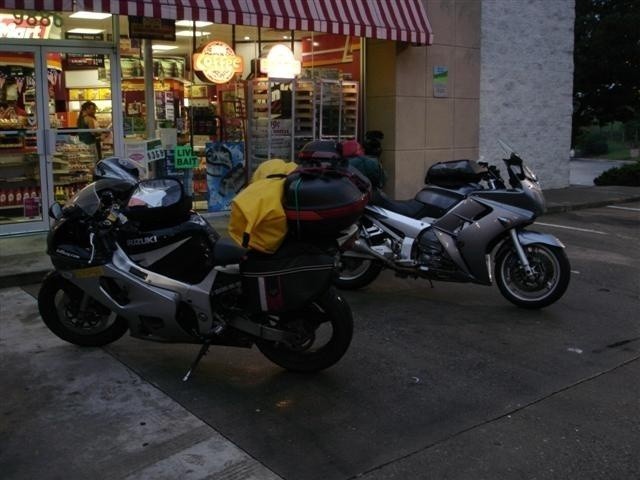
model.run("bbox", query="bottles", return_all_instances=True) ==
[0,181,86,207]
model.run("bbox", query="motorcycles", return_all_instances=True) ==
[297,139,571,309]
[38,158,372,383]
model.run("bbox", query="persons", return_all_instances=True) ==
[1,93,39,143]
[76,100,103,161]
[91,102,110,159]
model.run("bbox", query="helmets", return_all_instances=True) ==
[92,156,141,188]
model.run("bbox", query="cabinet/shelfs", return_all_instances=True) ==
[1,38,359,216]
